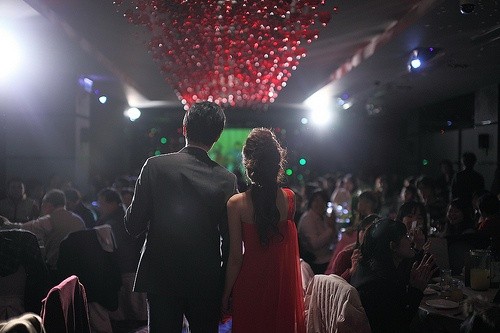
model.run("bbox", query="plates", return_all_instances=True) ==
[425,299,458,309]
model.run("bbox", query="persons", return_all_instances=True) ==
[125,100,238,333]
[0,176,146,276]
[291,152,500,333]
[220,128,308,333]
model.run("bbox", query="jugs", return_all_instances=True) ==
[470,250,496,291]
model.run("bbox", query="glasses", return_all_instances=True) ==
[395,232,410,245]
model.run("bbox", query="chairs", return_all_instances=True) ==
[0,219,149,333]
[304,274,372,333]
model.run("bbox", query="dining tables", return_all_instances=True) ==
[416,275,500,330]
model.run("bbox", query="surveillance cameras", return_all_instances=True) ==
[459,0,476,15]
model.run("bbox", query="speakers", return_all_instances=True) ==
[80,127,90,143]
[478,134,489,149]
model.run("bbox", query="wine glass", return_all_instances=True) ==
[456,288,464,306]
